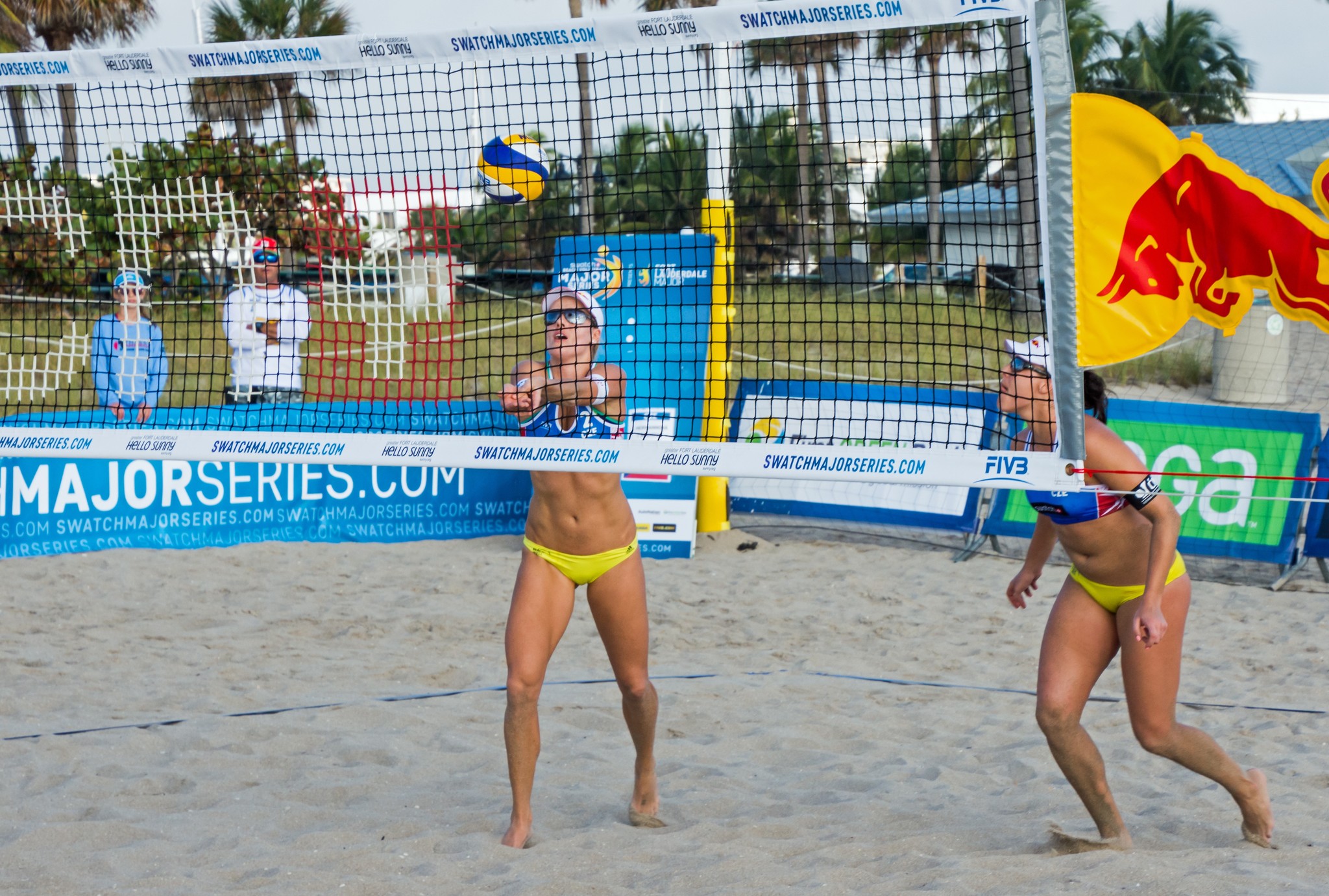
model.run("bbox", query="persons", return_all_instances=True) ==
[497,287,658,849]
[221,236,312,408]
[92,268,168,425]
[998,332,1272,850]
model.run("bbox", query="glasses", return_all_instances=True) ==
[116,288,144,296]
[1009,356,1052,379]
[254,255,278,263]
[544,308,598,329]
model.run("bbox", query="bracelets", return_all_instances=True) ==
[1122,474,1162,511]
[585,373,609,405]
[516,378,528,388]
[254,322,263,331]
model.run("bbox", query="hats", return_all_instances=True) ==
[112,271,144,288]
[541,285,604,333]
[1004,336,1052,377]
[252,236,281,258]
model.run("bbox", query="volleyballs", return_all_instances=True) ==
[477,133,551,206]
